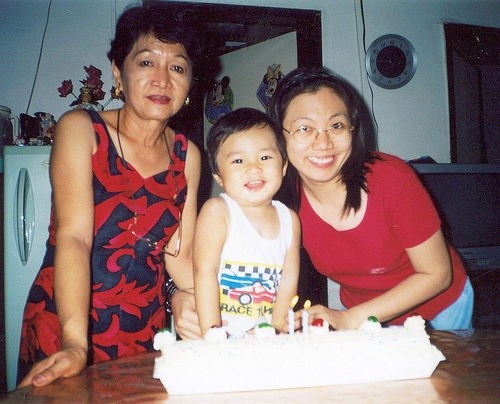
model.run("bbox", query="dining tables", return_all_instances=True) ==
[0,327,500,404]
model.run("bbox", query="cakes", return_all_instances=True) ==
[152,313,433,395]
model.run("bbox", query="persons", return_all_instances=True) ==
[192,107,301,338]
[15,5,201,390]
[171,65,474,340]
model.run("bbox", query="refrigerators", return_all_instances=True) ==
[4,145,55,393]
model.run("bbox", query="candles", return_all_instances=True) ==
[287,295,299,335]
[301,299,312,335]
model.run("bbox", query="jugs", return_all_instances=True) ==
[0,105,20,145]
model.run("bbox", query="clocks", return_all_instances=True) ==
[365,34,418,89]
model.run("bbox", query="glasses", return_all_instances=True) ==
[127,206,182,257]
[282,125,355,144]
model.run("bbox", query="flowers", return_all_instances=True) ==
[57,64,120,107]
